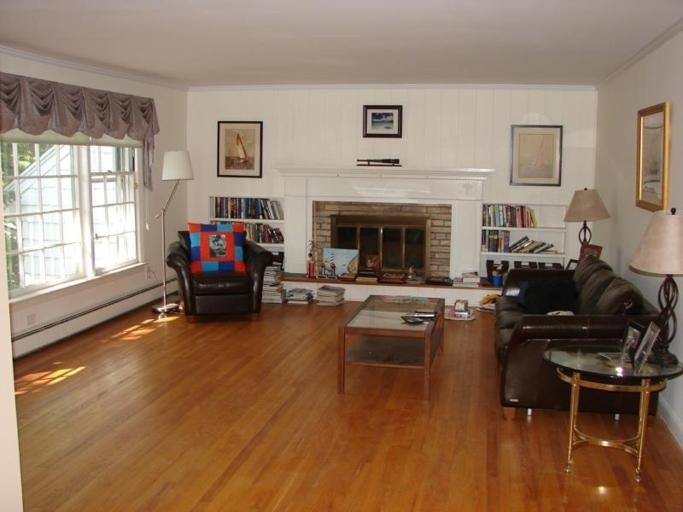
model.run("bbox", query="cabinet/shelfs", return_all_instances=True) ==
[208,196,284,268]
[479,201,567,278]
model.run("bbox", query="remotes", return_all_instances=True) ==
[403,312,436,318]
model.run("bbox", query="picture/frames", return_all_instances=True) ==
[565,243,603,272]
[361,104,403,139]
[621,319,661,370]
[216,121,263,178]
[634,100,670,214]
[508,124,563,187]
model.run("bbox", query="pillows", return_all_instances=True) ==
[187,221,247,275]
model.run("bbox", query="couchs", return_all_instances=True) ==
[493,254,666,414]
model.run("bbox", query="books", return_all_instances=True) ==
[351,267,481,290]
[478,203,559,255]
[403,308,437,319]
[208,194,346,306]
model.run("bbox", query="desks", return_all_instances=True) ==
[541,338,683,481]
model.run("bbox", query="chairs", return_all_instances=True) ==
[165,230,274,318]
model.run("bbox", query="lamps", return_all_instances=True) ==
[565,188,609,248]
[629,209,683,368]
[151,150,195,314]
